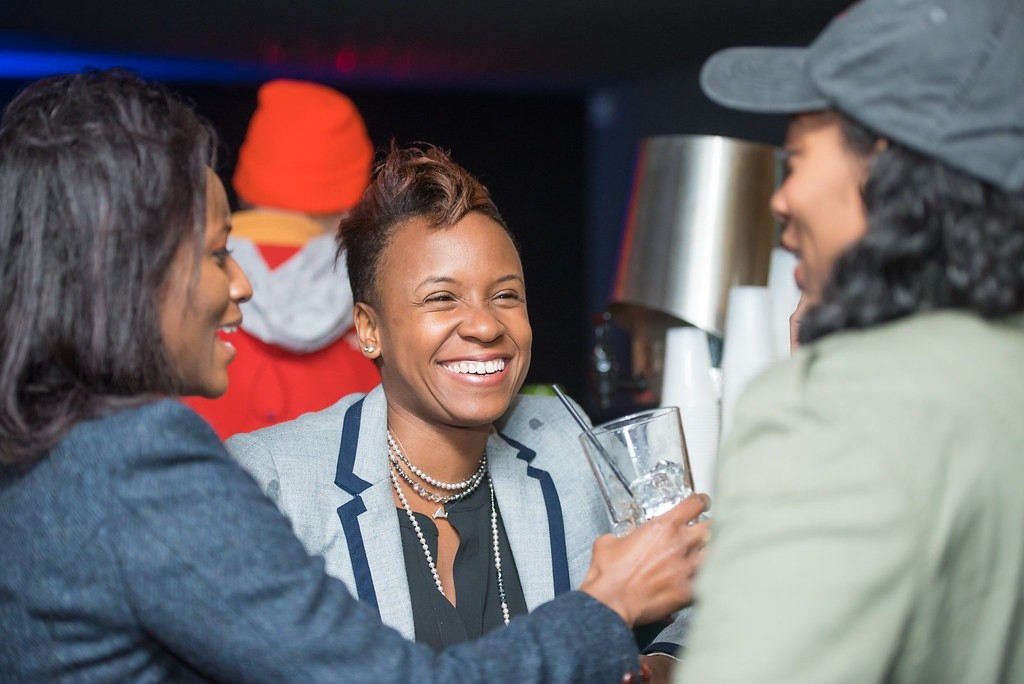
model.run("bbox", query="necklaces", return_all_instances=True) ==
[389,464,510,628]
[387,443,489,503]
[387,431,485,491]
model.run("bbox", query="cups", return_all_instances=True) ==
[658,285,775,488]
[578,407,692,536]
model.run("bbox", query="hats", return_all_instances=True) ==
[232,80,375,213]
[696,0,1024,196]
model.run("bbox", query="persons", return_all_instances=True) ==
[0,0,1023,684]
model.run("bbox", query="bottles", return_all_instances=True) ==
[594,316,620,389]
